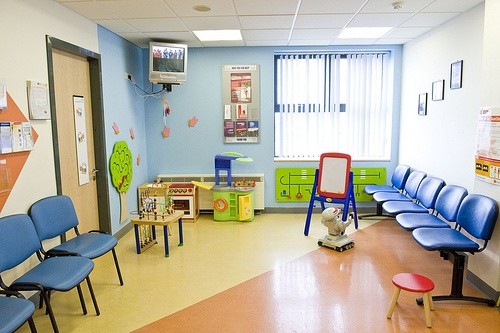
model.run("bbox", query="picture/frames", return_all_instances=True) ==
[418,93,428,116]
[432,79,445,101]
[449,60,463,89]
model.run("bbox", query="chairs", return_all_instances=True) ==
[28,195,124,315]
[0,290,38,333]
[358,165,498,308]
[0,214,100,333]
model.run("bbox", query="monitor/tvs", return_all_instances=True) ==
[148,42,187,84]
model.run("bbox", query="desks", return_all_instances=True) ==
[130,210,184,257]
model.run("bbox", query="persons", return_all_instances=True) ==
[153,48,182,59]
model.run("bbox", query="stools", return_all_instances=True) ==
[387,272,435,328]
